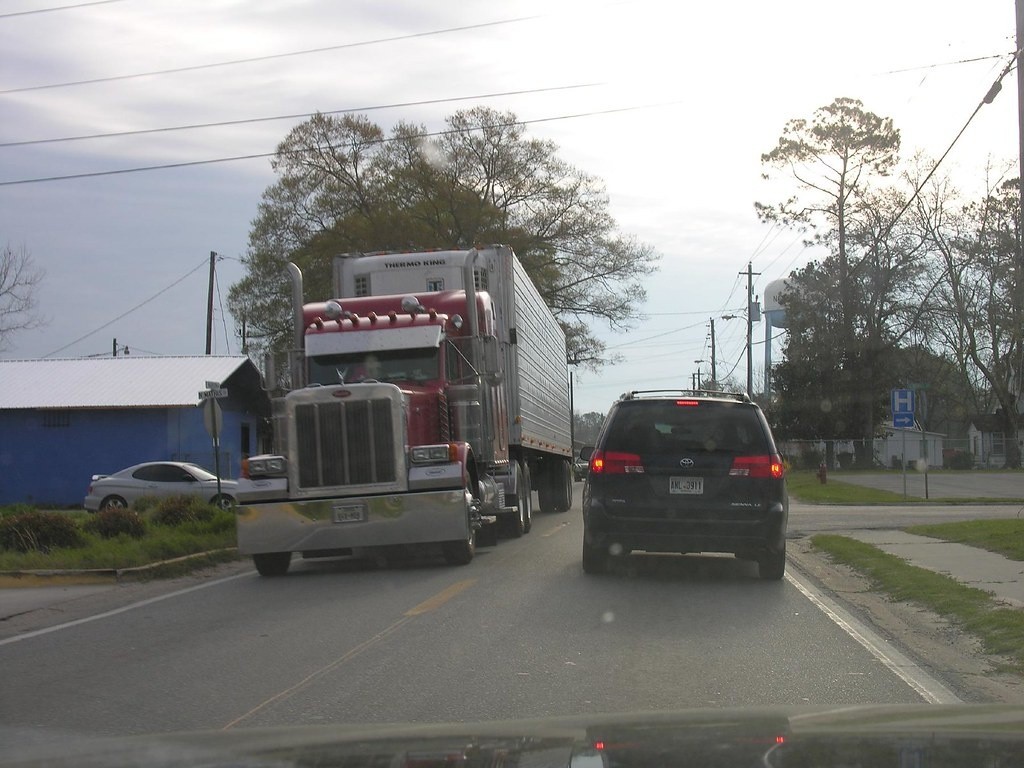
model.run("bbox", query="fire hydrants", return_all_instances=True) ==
[816,463,827,483]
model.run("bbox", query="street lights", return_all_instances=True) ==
[694,319,716,380]
[721,265,762,400]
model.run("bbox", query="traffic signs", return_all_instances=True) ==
[891,389,915,428]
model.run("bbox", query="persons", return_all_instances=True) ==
[241,453,250,478]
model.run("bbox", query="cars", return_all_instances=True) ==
[574,457,588,482]
[84,461,238,512]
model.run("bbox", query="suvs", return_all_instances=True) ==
[580,389,789,579]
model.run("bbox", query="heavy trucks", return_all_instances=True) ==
[235,243,574,576]
